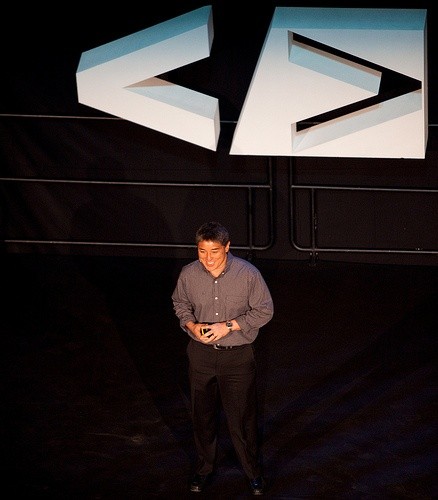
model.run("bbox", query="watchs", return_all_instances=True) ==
[226,321,233,332]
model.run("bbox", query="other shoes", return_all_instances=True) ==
[246,479,265,497]
[189,473,209,493]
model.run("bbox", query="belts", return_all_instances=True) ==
[198,341,249,352]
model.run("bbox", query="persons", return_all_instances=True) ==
[172,221,274,495]
